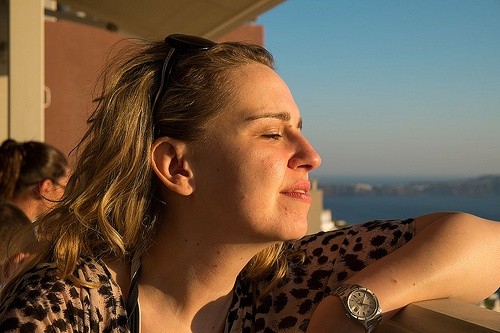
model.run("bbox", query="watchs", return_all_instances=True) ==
[329,282,385,333]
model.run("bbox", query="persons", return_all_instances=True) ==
[1,139,71,282]
[0,33,499,333]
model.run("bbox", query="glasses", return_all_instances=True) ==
[151,34,218,119]
